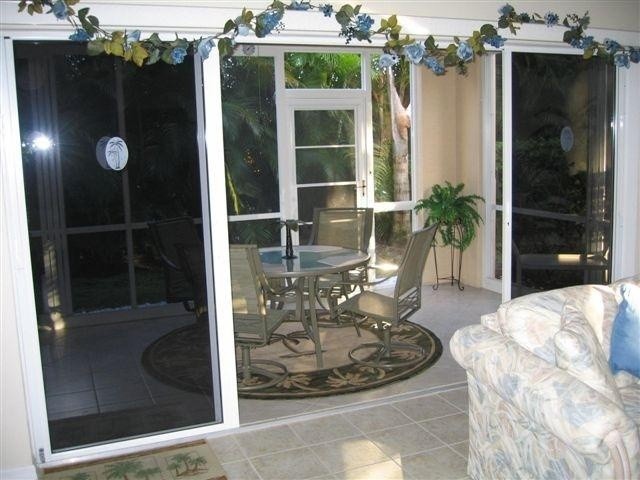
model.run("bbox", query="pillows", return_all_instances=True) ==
[608,283,640,379]
[554,298,625,410]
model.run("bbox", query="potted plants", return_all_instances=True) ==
[413,180,487,251]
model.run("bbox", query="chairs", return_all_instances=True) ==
[229,244,290,391]
[147,215,207,303]
[293,208,374,328]
[327,223,438,368]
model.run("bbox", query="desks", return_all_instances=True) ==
[258,245,371,368]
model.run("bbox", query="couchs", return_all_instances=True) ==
[450,275,640,480]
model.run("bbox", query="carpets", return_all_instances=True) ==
[141,310,442,400]
[37,437,230,480]
